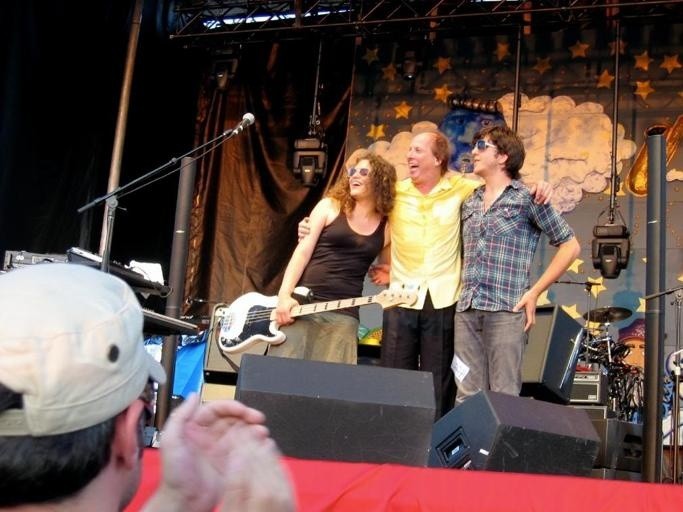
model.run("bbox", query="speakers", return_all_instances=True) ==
[519,303,585,407]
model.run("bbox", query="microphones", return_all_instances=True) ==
[232,111,256,136]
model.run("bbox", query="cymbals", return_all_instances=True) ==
[583,306,633,323]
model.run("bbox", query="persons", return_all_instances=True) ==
[264,147,392,366]
[1,259,297,510]
[450,127,581,407]
[295,125,551,422]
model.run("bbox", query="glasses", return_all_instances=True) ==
[469,139,506,151]
[349,168,379,177]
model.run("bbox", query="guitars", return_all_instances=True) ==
[218,285,419,353]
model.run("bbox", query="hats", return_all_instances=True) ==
[0,258,170,443]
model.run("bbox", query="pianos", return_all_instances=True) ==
[143,307,199,335]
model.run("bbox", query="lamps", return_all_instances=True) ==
[591,2,631,279]
[288,36,329,188]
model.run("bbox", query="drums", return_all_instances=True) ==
[619,318,647,369]
